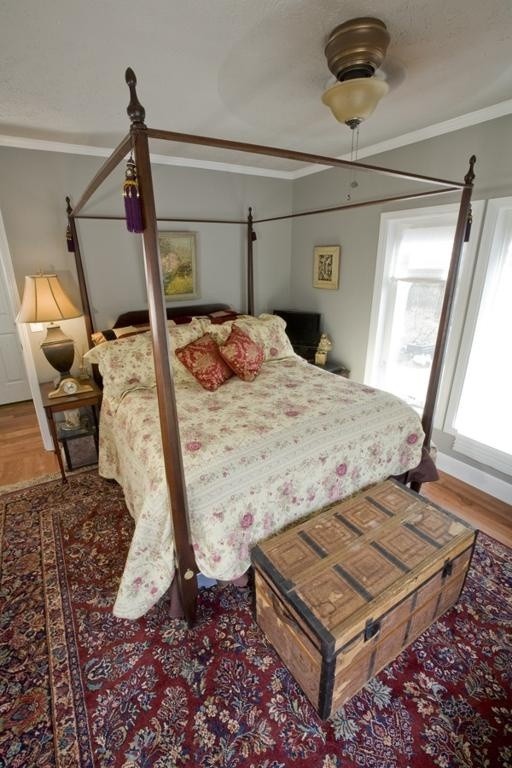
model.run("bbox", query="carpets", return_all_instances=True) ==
[2,462,512,765]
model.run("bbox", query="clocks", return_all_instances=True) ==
[47,377,94,399]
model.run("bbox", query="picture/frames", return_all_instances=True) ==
[155,229,199,301]
[312,244,342,290]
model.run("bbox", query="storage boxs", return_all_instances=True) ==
[249,475,481,722]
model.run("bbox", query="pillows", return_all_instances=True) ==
[86,310,294,392]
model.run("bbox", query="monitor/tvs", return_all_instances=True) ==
[274,310,321,349]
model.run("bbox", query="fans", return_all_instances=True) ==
[215,0,512,143]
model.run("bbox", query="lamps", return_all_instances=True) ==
[321,14,394,203]
[14,268,87,394]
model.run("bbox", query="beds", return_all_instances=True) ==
[62,63,481,630]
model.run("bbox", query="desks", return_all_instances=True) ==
[307,355,351,378]
[37,376,102,484]
